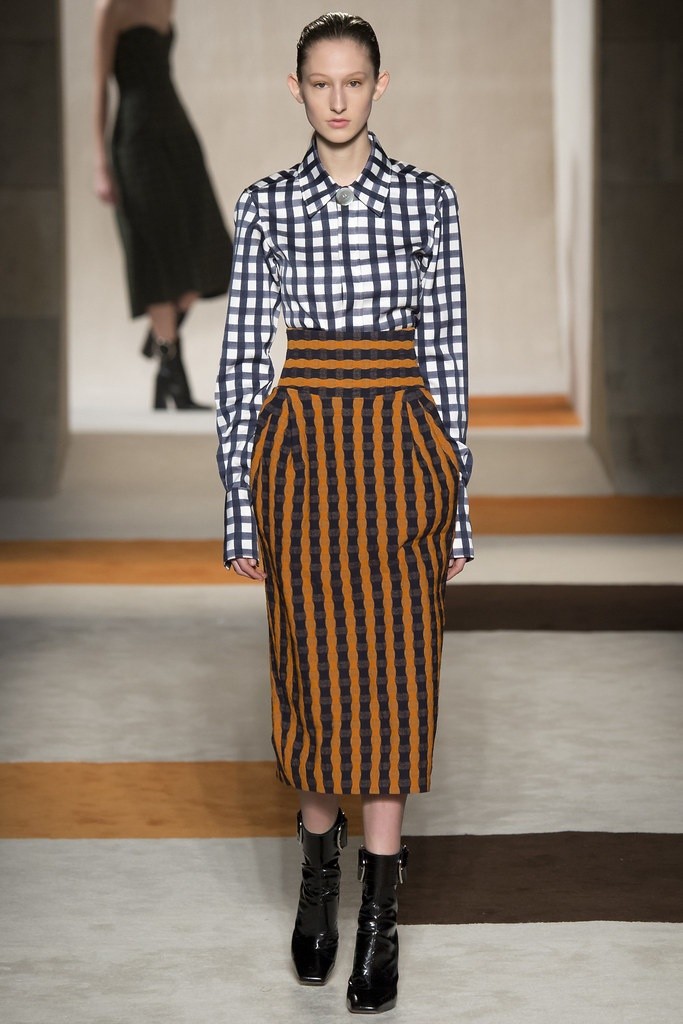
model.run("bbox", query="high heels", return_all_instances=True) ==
[143,307,184,357]
[153,339,212,410]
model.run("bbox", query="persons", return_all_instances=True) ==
[89,0,238,415]
[213,10,475,1014]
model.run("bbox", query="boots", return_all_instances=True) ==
[346,844,411,1016]
[291,806,347,985]
